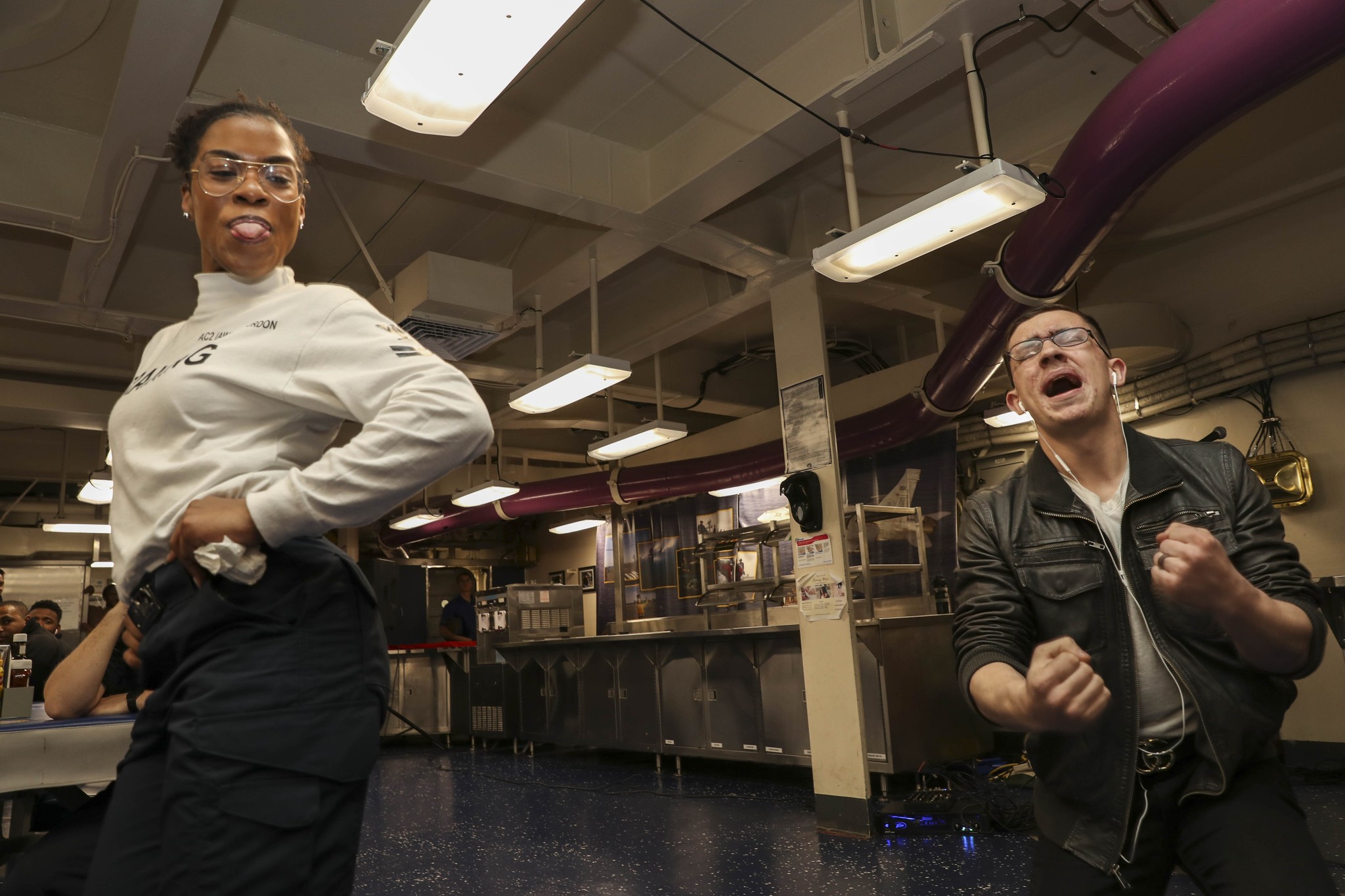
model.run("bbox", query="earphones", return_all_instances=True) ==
[1019,401,1026,413]
[1111,371,1117,386]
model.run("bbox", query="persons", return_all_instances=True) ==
[0,571,155,830]
[820,587,830,599]
[0,99,496,896]
[950,306,1339,896]
[713,525,716,533]
[439,572,477,642]
[582,572,592,587]
[698,521,706,541]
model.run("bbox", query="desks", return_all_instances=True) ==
[0,700,140,805]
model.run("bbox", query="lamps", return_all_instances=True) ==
[359,0,588,139]
[980,395,1039,430]
[503,255,638,419]
[386,443,523,533]
[41,446,116,572]
[546,507,609,536]
[581,354,691,464]
[807,30,1050,286]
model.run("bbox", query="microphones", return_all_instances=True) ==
[1198,426,1226,442]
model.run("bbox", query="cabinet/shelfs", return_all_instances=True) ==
[696,500,933,631]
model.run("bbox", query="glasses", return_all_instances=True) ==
[183,155,309,203]
[1004,327,1111,389]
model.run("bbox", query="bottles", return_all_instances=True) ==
[9,634,32,688]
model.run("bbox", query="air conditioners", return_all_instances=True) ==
[349,247,517,369]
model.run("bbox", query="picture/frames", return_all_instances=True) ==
[578,565,596,593]
[548,570,566,585]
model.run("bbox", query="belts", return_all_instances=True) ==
[126,572,167,636]
[1134,731,1199,784]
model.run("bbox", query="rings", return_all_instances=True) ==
[1157,554,1171,570]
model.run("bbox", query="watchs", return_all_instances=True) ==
[125,688,144,715]
